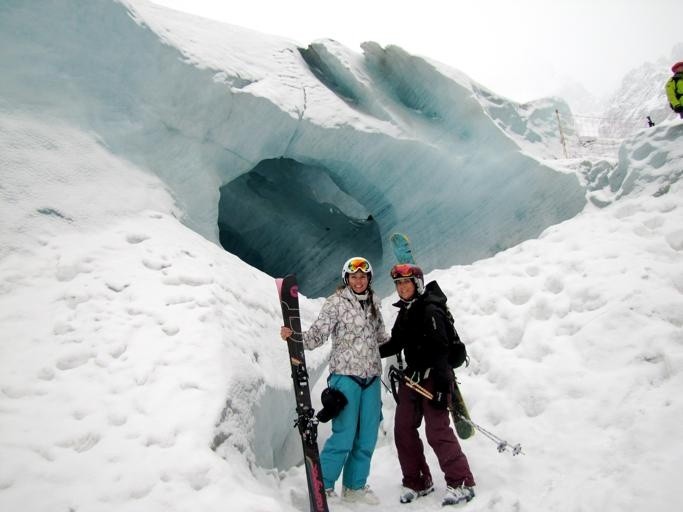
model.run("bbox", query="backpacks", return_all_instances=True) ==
[413,300,471,368]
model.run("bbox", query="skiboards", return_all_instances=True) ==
[275,274,330,512]
[390,233,475,439]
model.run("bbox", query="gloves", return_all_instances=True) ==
[429,385,449,411]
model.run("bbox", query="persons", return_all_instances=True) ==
[378,263,477,508]
[280,256,394,505]
[665,61,683,119]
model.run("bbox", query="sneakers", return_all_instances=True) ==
[340,485,381,506]
[324,488,342,506]
[399,485,434,505]
[441,483,475,506]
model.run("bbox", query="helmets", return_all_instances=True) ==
[392,262,425,294]
[341,256,373,284]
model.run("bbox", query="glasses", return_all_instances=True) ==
[348,258,370,271]
[390,264,416,278]
